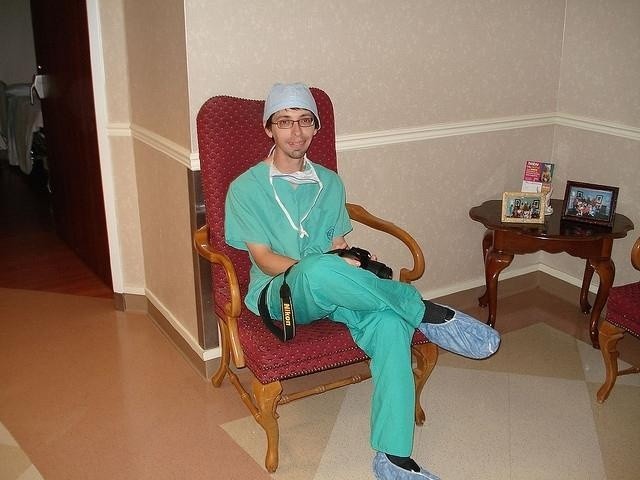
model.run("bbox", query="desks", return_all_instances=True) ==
[468,196,635,350]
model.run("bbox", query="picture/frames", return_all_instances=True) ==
[500,189,548,226]
[560,178,622,230]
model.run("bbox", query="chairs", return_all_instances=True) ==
[193,88,441,474]
[595,236,640,404]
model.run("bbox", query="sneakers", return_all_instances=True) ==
[421,301,499,360]
[374,452,438,479]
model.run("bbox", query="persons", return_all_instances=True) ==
[223,82,503,480]
[509,201,537,218]
[574,192,602,217]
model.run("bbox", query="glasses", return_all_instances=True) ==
[272,118,314,128]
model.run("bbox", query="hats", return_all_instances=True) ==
[263,83,321,130]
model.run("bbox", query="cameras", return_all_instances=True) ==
[349,246,393,280]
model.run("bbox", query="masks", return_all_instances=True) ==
[271,164,320,185]
